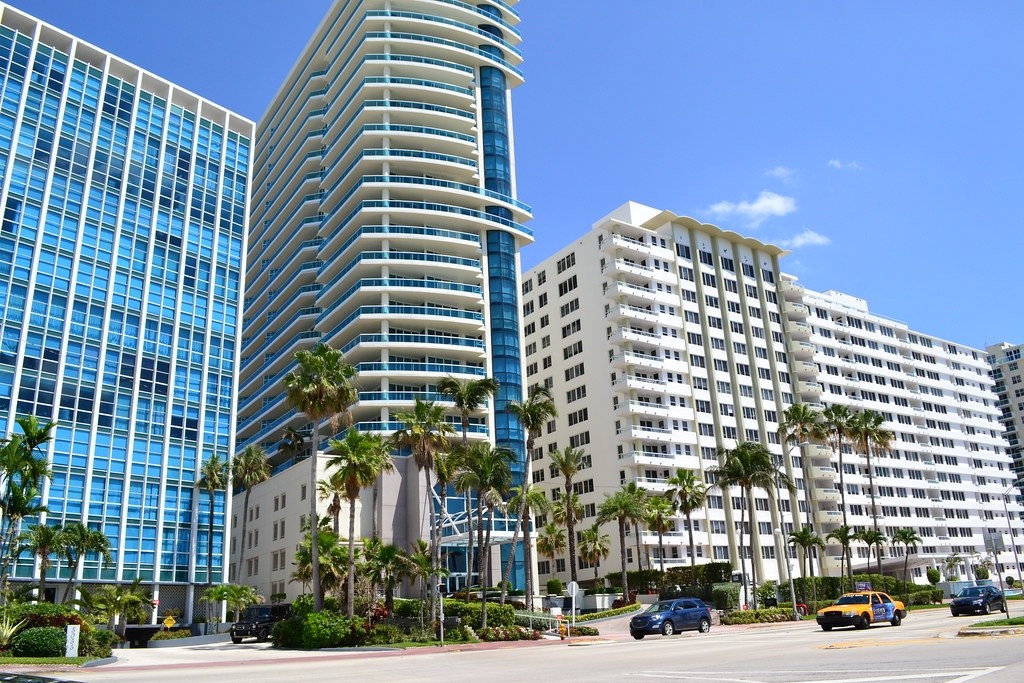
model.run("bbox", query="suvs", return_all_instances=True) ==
[230,602,294,644]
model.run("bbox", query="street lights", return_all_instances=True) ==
[1003,478,1024,596]
[773,441,810,621]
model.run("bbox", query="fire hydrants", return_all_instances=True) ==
[558,623,566,641]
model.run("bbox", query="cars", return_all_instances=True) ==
[630,598,712,640]
[816,590,907,632]
[949,585,1006,617]
